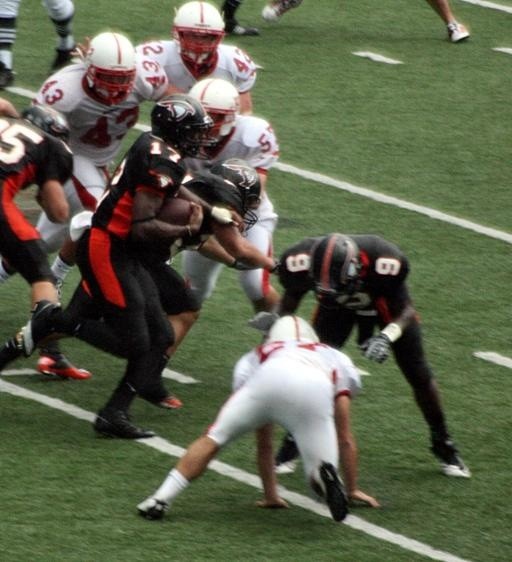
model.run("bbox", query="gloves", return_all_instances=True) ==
[231,256,259,271]
[247,311,280,332]
[359,331,392,364]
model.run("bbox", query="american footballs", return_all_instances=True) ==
[156,198,193,225]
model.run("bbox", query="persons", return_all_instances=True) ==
[138,316,382,526]
[262,1,472,43]
[0,0,283,438]
[245,234,471,484]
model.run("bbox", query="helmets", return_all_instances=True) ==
[186,78,240,147]
[311,232,363,309]
[84,32,136,101]
[21,104,71,145]
[172,1,226,66]
[150,94,213,155]
[209,157,262,229]
[265,314,320,343]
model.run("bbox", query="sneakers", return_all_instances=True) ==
[0,331,30,370]
[316,460,352,524]
[441,458,475,480]
[48,45,80,75]
[446,21,470,43]
[220,18,260,37]
[133,384,183,410]
[93,412,157,440]
[0,60,13,89]
[50,273,65,302]
[36,356,93,381]
[271,458,298,475]
[134,496,170,520]
[260,0,302,23]
